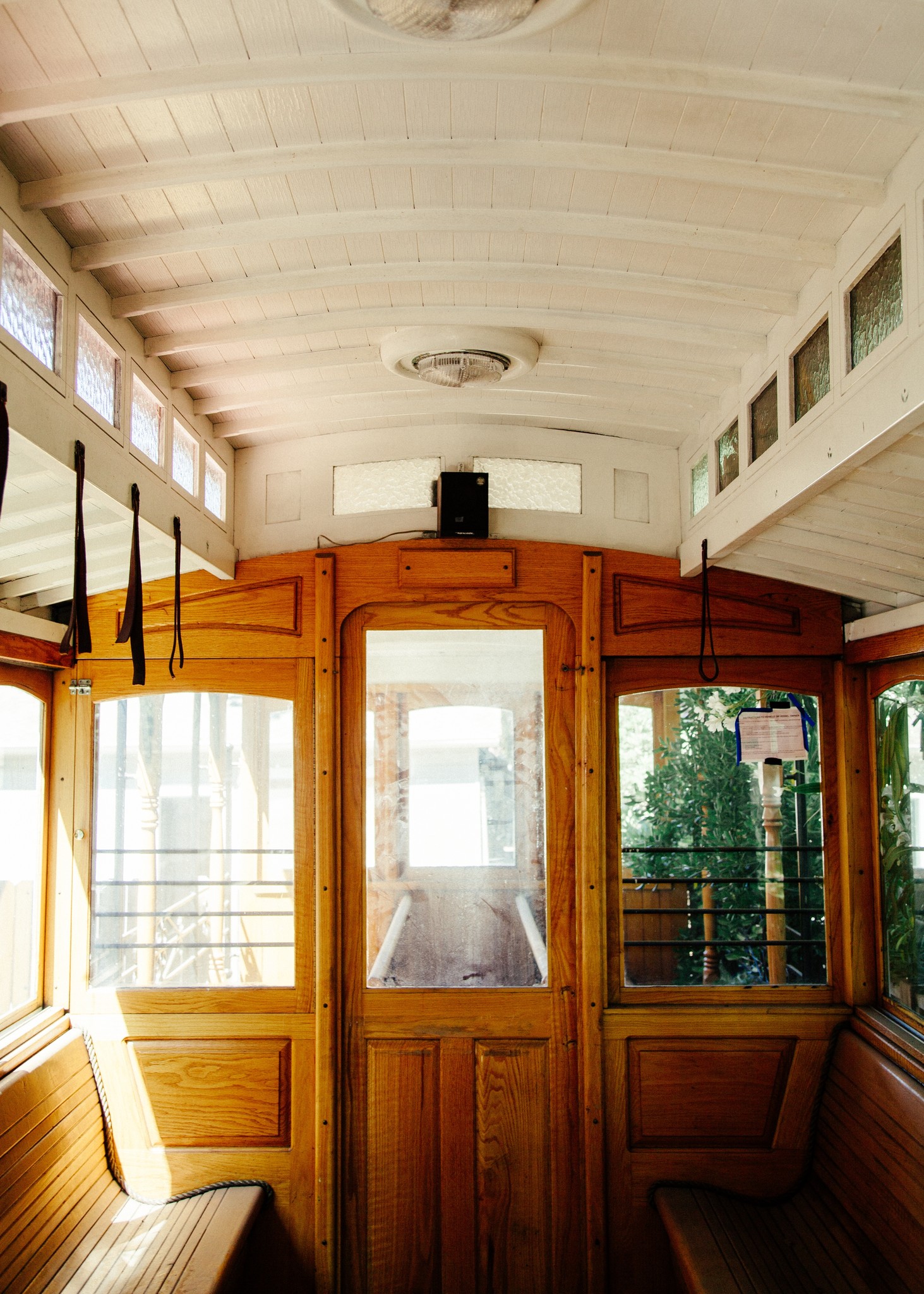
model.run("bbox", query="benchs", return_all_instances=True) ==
[1,1030,275,1294]
[644,1021,923,1294]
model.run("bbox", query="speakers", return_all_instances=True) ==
[437,472,489,539]
